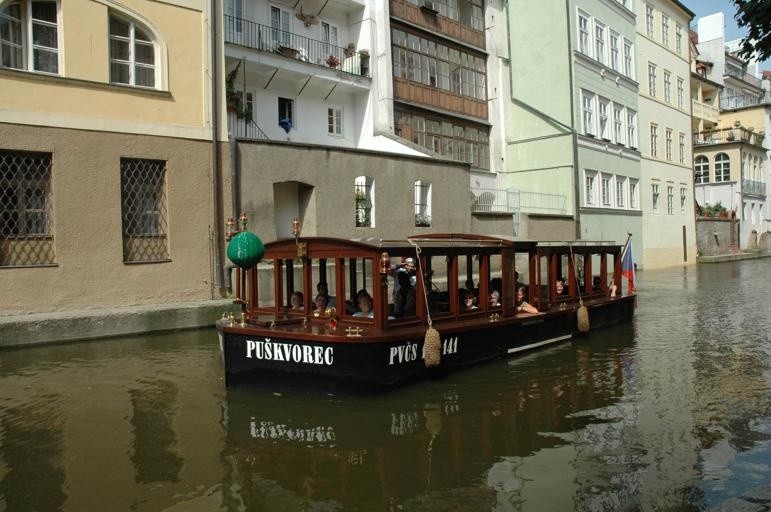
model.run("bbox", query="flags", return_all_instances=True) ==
[621,239,636,292]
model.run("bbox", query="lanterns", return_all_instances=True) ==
[228,229,267,272]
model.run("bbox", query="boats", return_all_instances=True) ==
[215,323,635,509]
[209,210,637,398]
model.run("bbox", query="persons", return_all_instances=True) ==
[389,257,441,316]
[290,291,304,310]
[544,276,601,297]
[312,281,336,313]
[459,271,538,314]
[345,290,373,317]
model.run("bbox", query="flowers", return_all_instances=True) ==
[324,55,341,65]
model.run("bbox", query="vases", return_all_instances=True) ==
[329,65,336,69]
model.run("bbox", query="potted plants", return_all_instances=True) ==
[703,205,728,219]
